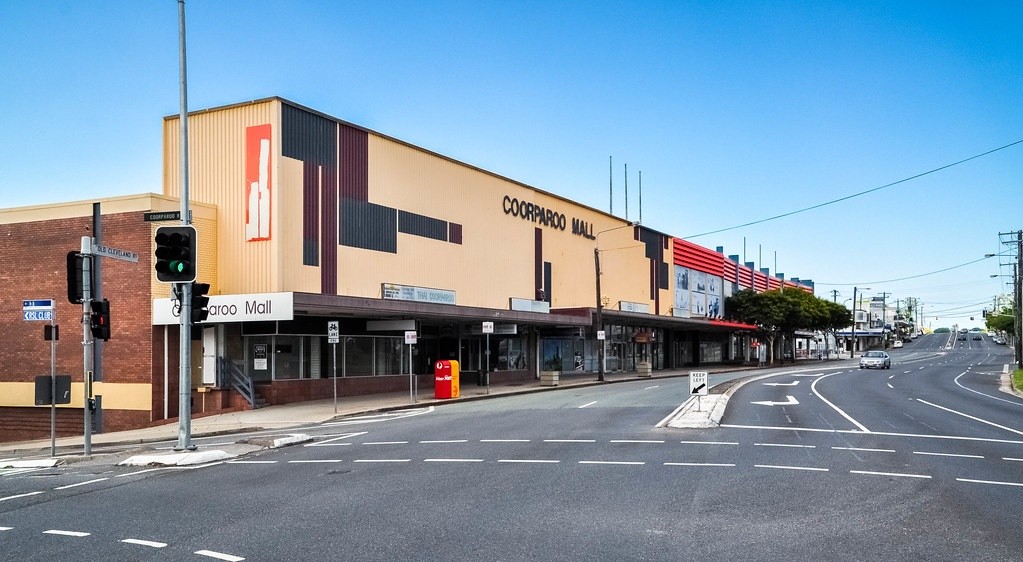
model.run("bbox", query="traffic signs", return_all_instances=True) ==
[688,370,709,396]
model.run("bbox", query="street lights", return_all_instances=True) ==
[594,221,641,380]
[984,253,1023,370]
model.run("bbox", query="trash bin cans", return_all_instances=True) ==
[434,360,451,398]
[449,360,460,398]
[478,370,487,386]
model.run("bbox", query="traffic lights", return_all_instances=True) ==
[89,300,111,342]
[155,225,198,284]
[192,280,211,324]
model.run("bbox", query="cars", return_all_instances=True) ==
[957,327,1007,345]
[910,330,922,339]
[859,350,891,370]
[902,336,912,343]
[894,340,903,348]
